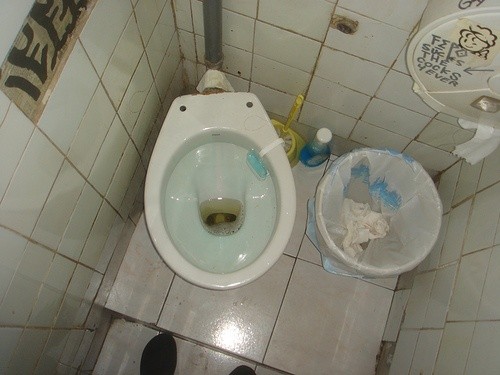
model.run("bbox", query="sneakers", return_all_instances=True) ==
[140,332,177,374]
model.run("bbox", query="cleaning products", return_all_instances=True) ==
[300,127,333,168]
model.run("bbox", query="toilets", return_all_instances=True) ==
[140,90,298,292]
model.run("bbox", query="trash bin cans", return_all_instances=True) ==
[315,149,443,278]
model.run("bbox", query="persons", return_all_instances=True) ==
[139,333,256,375]
